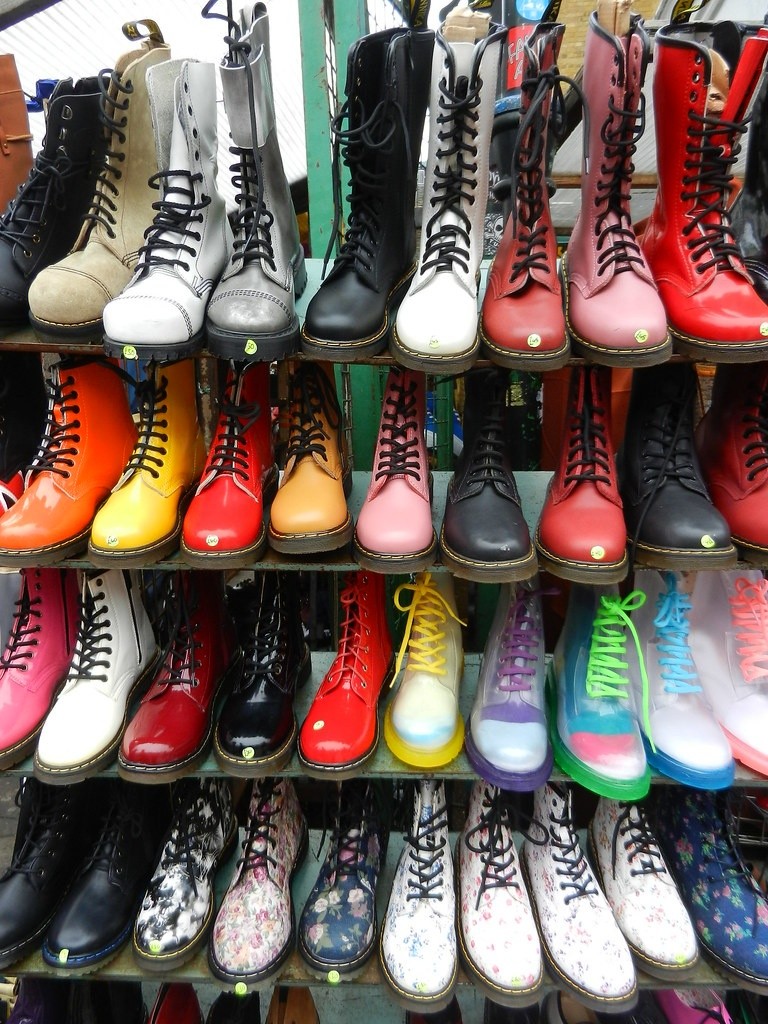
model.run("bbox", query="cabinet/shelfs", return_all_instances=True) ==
[0,257,768,990]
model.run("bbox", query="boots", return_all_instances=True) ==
[0,0,767,1024]
